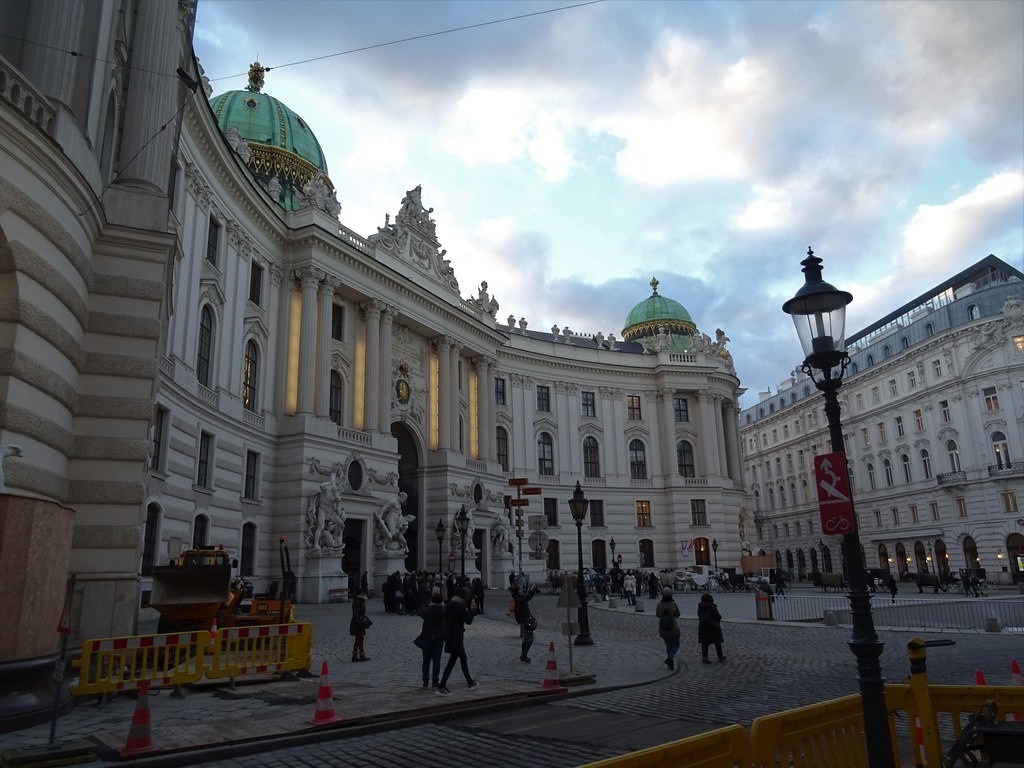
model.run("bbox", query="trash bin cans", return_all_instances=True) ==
[755,588,770,620]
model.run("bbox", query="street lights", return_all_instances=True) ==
[455,504,470,575]
[712,538,719,572]
[568,480,594,645]
[435,518,447,572]
[783,245,898,768]
[609,537,616,561]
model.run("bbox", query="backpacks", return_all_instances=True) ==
[659,600,674,631]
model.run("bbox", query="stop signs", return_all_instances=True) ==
[617,554,622,563]
[448,554,455,561]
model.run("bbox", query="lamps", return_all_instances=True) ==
[926,553,931,561]
[887,553,895,563]
[906,552,912,562]
[997,548,1002,559]
[975,556,982,562]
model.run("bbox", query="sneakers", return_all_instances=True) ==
[435,686,453,697]
[467,680,479,692]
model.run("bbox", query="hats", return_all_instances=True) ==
[456,589,466,599]
[509,585,520,595]
[429,592,443,604]
[661,586,672,598]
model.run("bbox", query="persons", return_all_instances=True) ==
[887,575,898,603]
[314,479,344,549]
[349,588,369,662]
[378,491,410,552]
[496,508,510,548]
[961,572,980,598]
[698,593,726,664]
[454,505,479,552]
[509,568,537,663]
[361,571,368,595]
[382,568,485,616]
[414,587,479,696]
[775,573,787,599]
[657,327,668,350]
[656,587,681,670]
[548,567,676,607]
[401,186,435,223]
[759,577,774,602]
[866,570,876,592]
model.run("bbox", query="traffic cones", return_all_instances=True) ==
[542,642,560,689]
[304,661,344,725]
[122,687,160,754]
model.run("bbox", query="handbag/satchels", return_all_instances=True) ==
[523,614,537,631]
[701,605,720,630]
[362,616,373,628]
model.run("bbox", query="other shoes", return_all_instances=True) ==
[703,660,711,664]
[964,593,979,597]
[664,656,674,670]
[719,656,726,662]
[892,600,895,603]
[649,597,656,599]
[620,595,624,599]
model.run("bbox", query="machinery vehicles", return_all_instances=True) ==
[148,543,296,649]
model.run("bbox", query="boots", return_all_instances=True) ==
[352,650,359,662]
[628,598,636,606]
[358,650,370,662]
[431,677,440,689]
[520,641,532,663]
[422,678,430,690]
